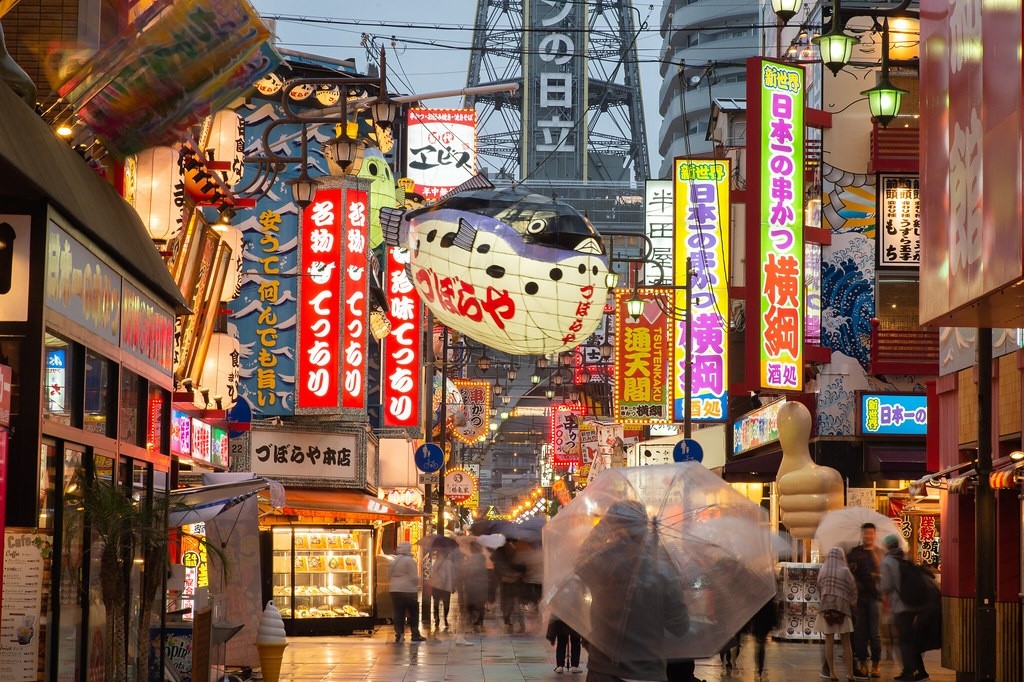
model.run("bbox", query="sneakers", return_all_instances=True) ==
[556,666,563,674]
[411,630,427,641]
[570,667,583,673]
[396,633,405,644]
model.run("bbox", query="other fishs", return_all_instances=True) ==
[378,172,611,354]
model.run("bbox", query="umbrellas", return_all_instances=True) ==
[416,533,458,551]
[689,516,798,561]
[540,460,778,662]
[467,515,546,542]
[814,506,910,559]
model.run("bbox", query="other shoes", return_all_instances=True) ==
[434,619,440,627]
[820,665,931,682]
[444,619,450,627]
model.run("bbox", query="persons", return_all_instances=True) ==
[555,618,581,673]
[817,546,871,682]
[576,501,699,682]
[387,541,426,642]
[706,558,782,680]
[846,523,884,677]
[428,536,544,647]
[875,531,930,682]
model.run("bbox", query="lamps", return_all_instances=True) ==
[181,379,223,412]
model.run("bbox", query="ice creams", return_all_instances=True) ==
[253,600,289,682]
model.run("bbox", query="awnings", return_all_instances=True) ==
[908,450,1024,496]
[160,473,285,526]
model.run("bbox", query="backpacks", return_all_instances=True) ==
[887,556,934,606]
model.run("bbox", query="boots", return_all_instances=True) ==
[456,634,473,647]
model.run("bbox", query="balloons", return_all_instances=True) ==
[379,172,610,356]
